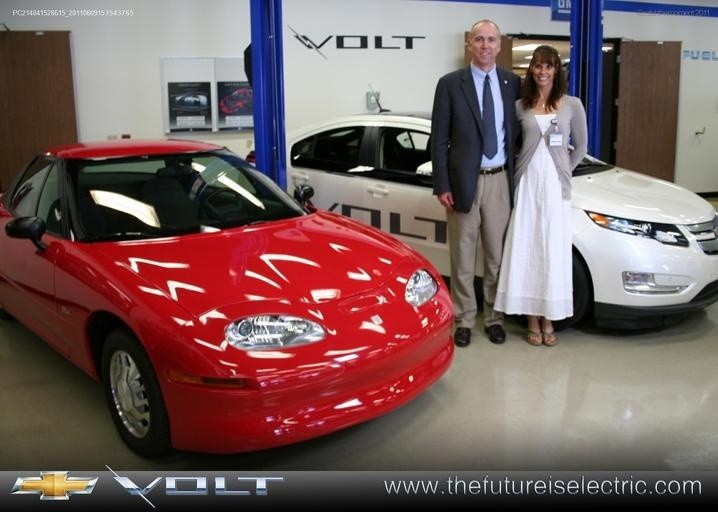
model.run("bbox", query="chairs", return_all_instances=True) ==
[133,177,201,236]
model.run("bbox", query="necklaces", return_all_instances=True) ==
[542,103,545,108]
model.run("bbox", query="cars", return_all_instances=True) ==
[220,87,252,113]
[245,110,717,335]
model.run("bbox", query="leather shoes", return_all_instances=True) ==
[454,327,479,347]
[485,325,505,344]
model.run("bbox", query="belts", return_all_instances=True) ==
[480,164,508,177]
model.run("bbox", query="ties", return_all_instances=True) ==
[483,73,498,160]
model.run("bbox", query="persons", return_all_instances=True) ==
[430,18,525,348]
[492,45,589,347]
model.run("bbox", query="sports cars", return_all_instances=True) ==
[2,139,454,462]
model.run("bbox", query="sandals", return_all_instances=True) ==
[524,327,542,346]
[542,330,559,346]
